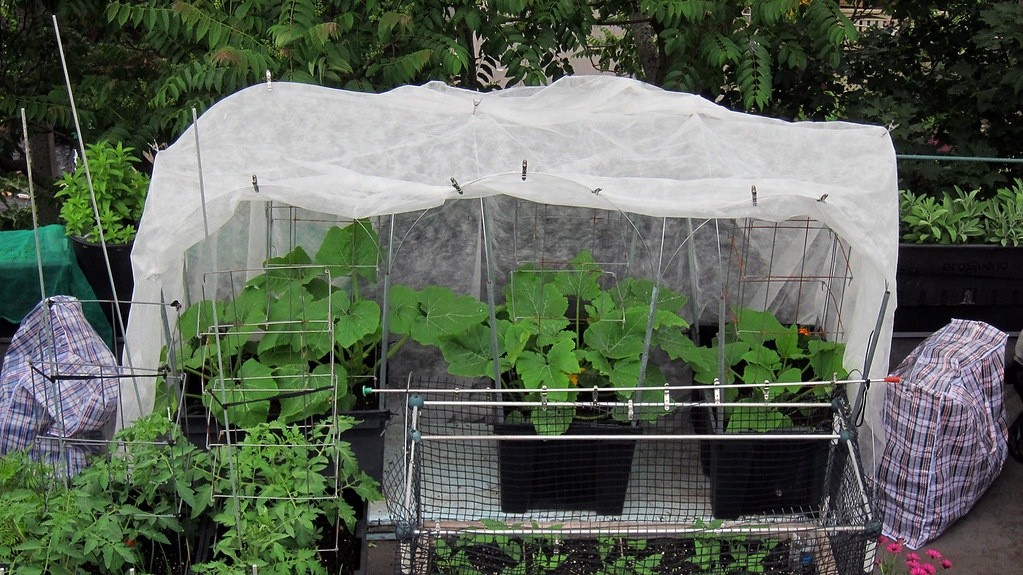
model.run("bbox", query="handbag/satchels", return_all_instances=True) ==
[0,295,122,480]
[867,319,1011,548]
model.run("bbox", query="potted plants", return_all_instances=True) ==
[52,131,1022,519]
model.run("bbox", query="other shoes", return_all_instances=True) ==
[1009,422,1023,463]
